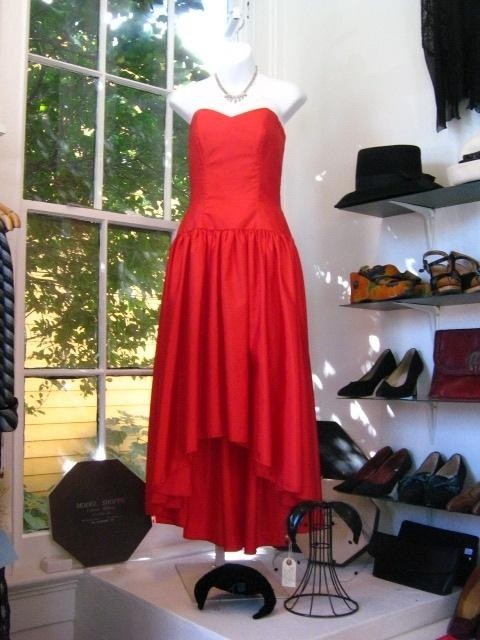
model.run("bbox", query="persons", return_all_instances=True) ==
[142,42,326,556]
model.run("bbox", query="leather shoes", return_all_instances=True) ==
[332,445,479,514]
[447,563,479,638]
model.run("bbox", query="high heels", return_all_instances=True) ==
[337,349,396,397]
[375,347,422,398]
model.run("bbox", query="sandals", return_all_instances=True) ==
[421,250,461,295]
[348,271,409,303]
[366,265,429,297]
[448,252,480,293]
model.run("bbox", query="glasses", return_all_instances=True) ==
[287,499,361,550]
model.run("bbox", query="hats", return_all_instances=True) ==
[194,563,277,617]
[447,138,480,188]
[333,144,441,205]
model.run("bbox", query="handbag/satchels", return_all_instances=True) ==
[371,537,463,597]
[397,522,479,589]
[429,327,480,398]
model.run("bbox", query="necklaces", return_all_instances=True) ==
[212,64,261,105]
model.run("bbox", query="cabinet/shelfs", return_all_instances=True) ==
[331,179,480,518]
